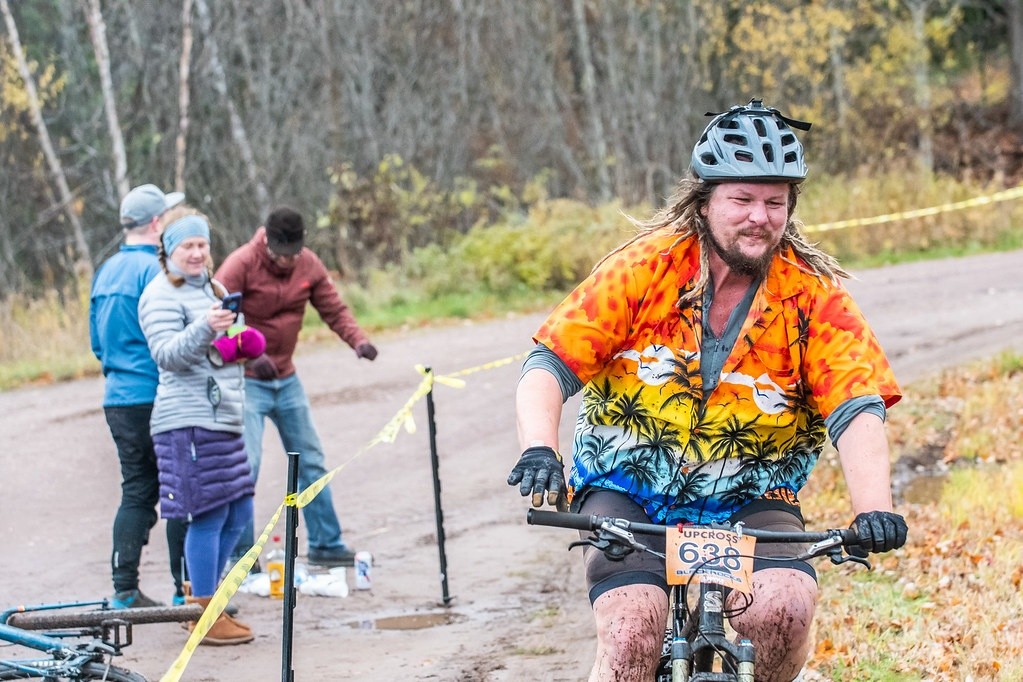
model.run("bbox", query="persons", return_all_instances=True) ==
[508,98,907,682]
[214,208,377,575]
[88,183,238,615]
[136,206,256,645]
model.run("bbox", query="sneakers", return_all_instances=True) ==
[171,593,185,606]
[111,587,164,610]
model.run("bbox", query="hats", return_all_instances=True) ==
[264,207,306,257]
[118,183,186,226]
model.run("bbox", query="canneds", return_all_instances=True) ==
[354,550,372,591]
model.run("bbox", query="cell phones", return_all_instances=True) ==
[223,292,242,324]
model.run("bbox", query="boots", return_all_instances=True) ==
[180,581,256,645]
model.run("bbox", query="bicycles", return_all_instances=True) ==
[0,598,238,682]
[525,508,874,682]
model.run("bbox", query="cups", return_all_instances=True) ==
[299,566,349,597]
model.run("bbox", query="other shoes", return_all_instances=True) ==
[306,544,378,567]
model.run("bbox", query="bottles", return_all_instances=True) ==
[266,536,285,597]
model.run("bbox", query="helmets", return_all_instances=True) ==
[689,96,813,184]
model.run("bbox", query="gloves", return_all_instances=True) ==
[248,353,280,381]
[843,511,908,558]
[354,343,379,360]
[507,445,569,513]
